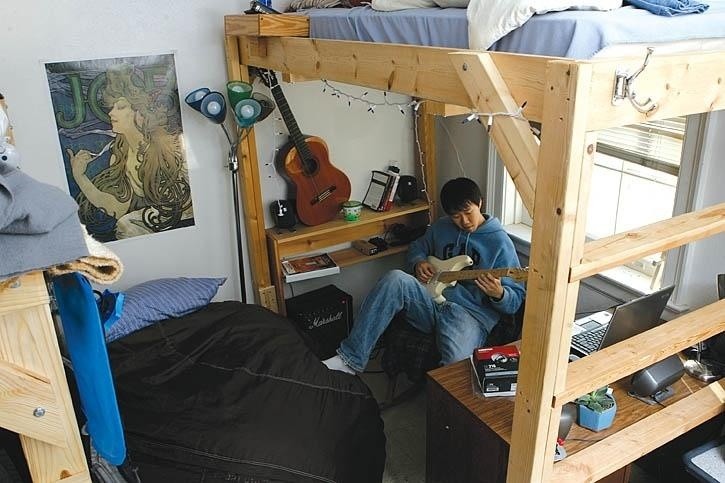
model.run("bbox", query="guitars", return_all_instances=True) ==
[256,67,352,226]
[413,255,529,303]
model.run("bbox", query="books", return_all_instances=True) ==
[362,166,400,212]
[281,252,340,283]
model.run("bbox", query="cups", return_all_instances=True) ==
[337,201,363,222]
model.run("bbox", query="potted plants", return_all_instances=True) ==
[577,386,619,433]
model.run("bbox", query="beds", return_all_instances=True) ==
[224,0,725,483]
[1,92,130,482]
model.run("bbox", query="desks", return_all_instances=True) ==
[265,196,430,315]
[425,300,724,483]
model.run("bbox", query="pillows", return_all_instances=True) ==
[103,277,230,348]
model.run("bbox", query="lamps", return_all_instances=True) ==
[184,81,276,304]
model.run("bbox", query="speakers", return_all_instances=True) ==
[397,175,417,208]
[285,284,353,361]
[270,200,296,234]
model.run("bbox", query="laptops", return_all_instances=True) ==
[570,285,675,358]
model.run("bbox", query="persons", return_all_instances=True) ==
[66,69,194,240]
[321,177,527,376]
